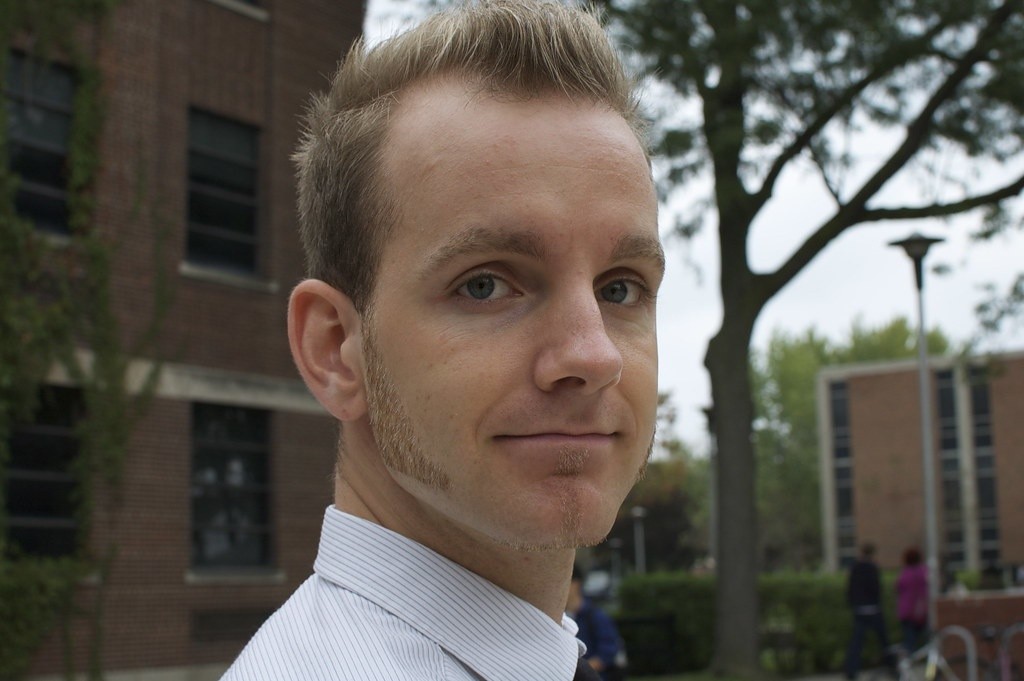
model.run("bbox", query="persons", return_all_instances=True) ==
[838,542,900,681]
[893,548,933,667]
[557,563,628,681]
[211,1,664,681]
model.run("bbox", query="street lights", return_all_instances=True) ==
[888,232,947,639]
